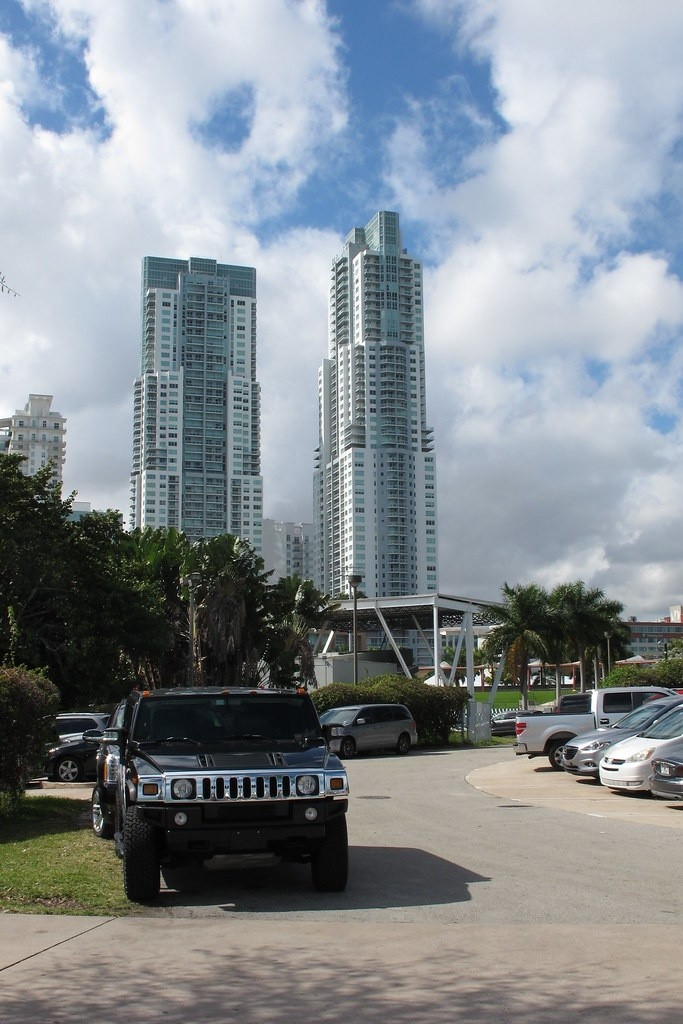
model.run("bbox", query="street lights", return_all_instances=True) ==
[603,631,614,675]
[185,571,201,688]
[345,573,366,687]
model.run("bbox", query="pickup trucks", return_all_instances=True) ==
[511,685,683,772]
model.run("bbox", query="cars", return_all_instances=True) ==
[42,732,99,782]
[559,692,683,783]
[599,704,683,792]
[646,734,683,801]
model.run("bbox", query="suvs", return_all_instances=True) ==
[306,703,418,760]
[37,712,112,751]
[472,709,544,736]
[78,684,349,902]
